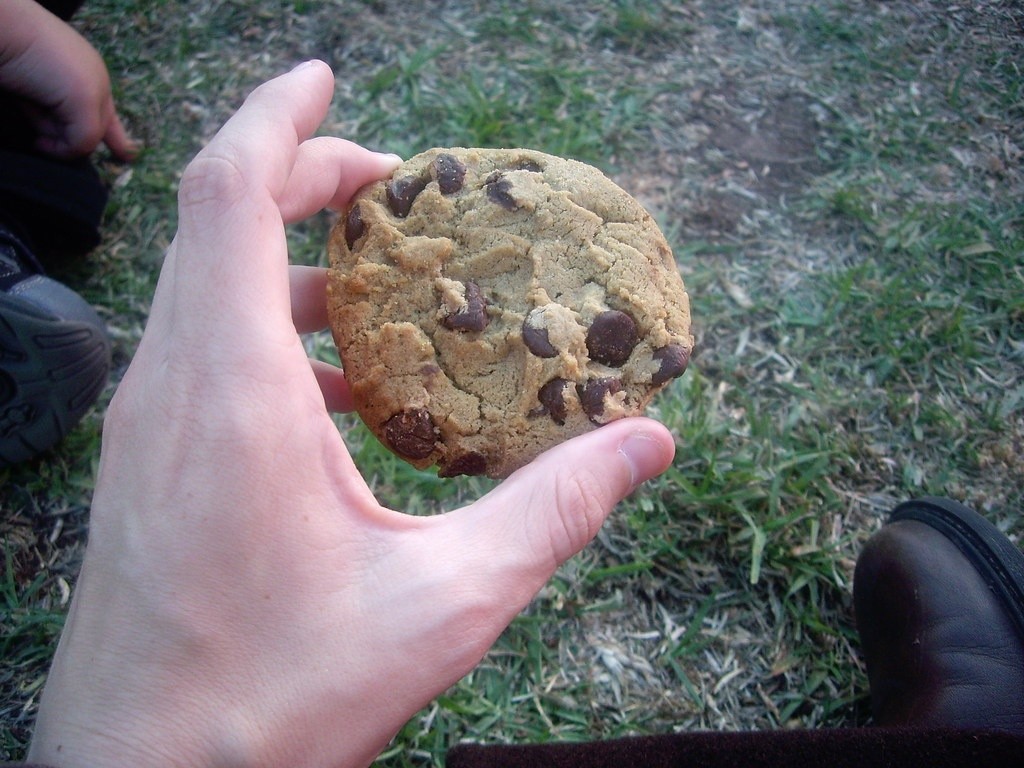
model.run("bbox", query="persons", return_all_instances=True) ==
[26,59,1024,768]
[1,1,146,507]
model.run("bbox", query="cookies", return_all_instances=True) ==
[326,147,693,480]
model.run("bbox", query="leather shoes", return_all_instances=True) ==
[853,496,1024,729]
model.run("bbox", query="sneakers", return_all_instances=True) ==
[0,231,113,466]
